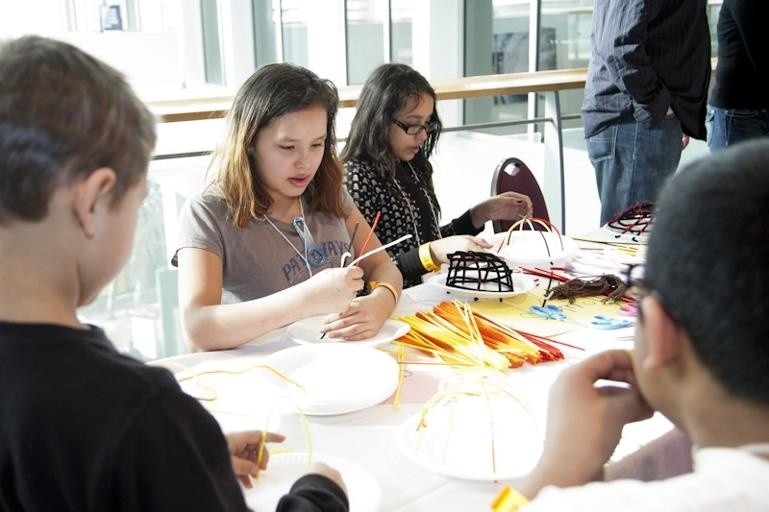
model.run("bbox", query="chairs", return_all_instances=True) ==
[492,158,552,234]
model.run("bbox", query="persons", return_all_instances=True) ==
[706,0,769,154]
[0,32,351,512]
[337,61,535,299]
[578,0,714,227]
[489,137,769,512]
[163,60,405,354]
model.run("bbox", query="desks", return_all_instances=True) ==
[139,215,654,512]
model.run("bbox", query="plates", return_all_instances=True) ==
[425,266,534,301]
[481,230,577,265]
[604,218,652,238]
[251,340,401,418]
[285,312,408,348]
[391,407,545,484]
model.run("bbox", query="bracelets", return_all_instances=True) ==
[490,484,530,512]
[418,241,441,273]
[368,280,399,304]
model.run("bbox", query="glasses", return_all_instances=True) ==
[392,119,437,135]
